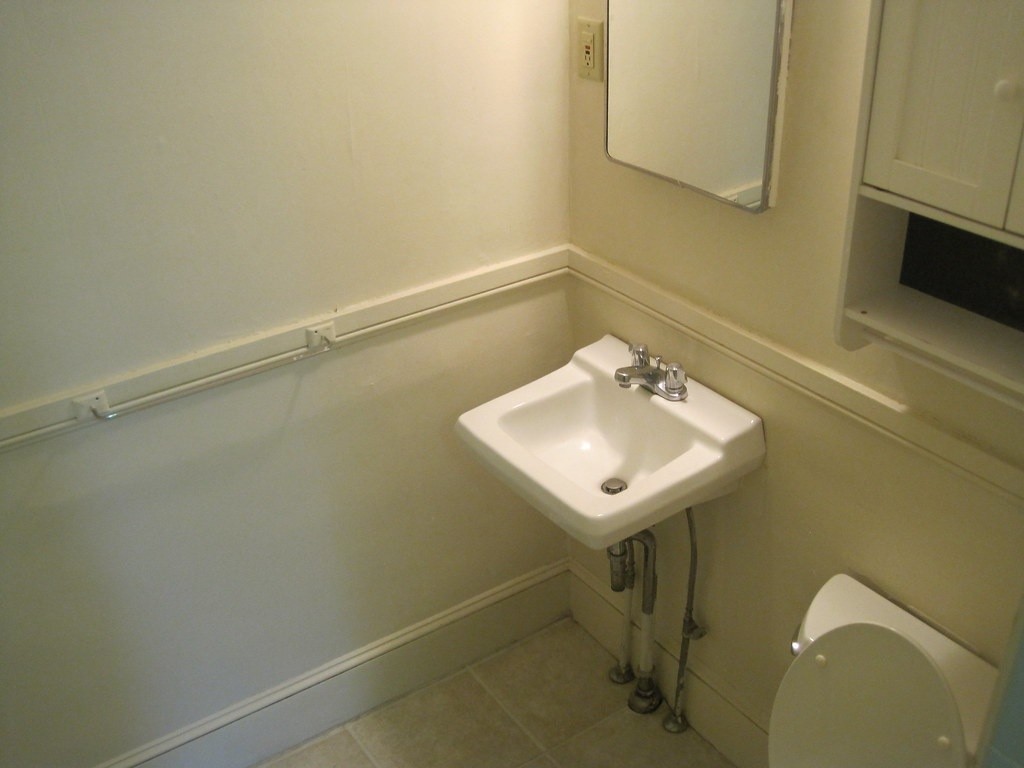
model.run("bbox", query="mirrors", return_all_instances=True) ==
[606,0,786,215]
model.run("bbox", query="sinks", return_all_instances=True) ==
[454,331,767,552]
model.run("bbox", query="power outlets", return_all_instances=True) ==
[576,17,604,82]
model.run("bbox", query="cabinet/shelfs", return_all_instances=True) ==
[834,1,1024,411]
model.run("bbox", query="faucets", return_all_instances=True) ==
[613,354,666,395]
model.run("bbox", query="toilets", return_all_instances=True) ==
[766,569,999,768]
